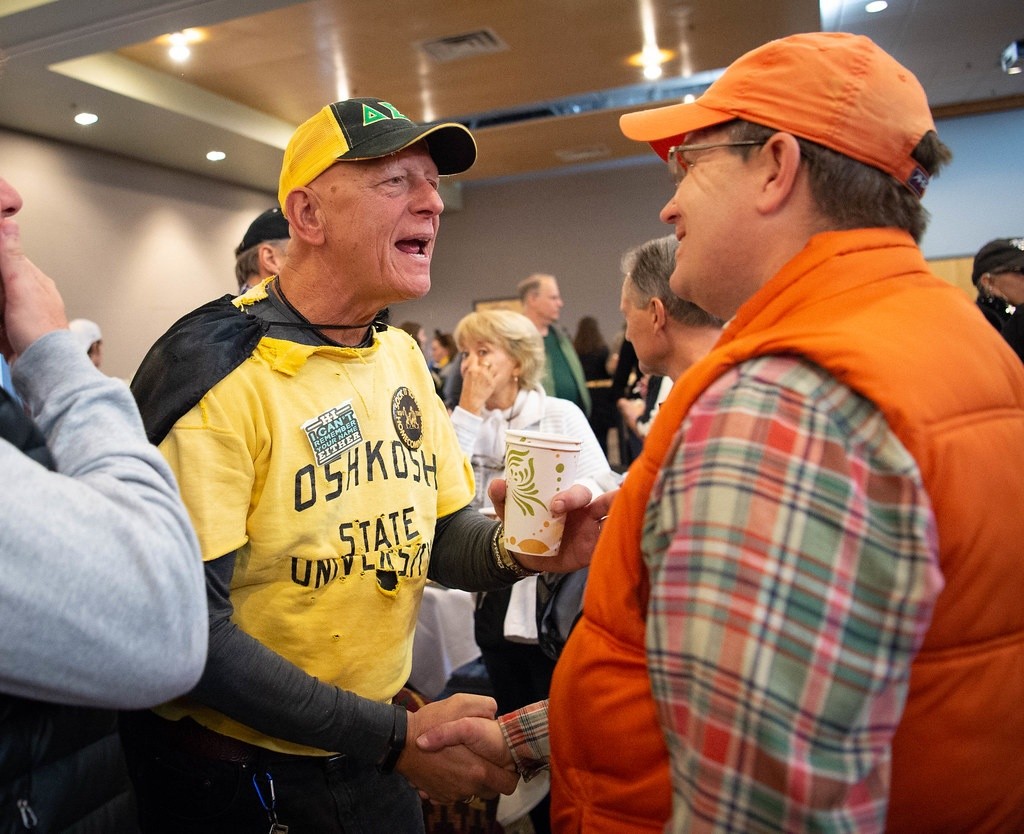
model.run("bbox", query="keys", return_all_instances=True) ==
[269,823,288,834]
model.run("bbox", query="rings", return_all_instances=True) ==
[463,794,474,805]
[597,520,602,532]
[482,361,489,366]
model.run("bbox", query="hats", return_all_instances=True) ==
[972,238,1024,285]
[278,97,477,219]
[235,205,292,259]
[618,31,942,199]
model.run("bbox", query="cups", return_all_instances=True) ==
[503,429,583,558]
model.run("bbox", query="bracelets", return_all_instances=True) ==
[491,522,517,575]
[498,527,534,576]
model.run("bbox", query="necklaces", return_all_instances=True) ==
[275,275,373,347]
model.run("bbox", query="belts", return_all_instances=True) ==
[257,756,347,779]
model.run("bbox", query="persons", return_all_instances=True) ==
[0,99,724,834]
[410,31,1024,834]
[972,235,1024,363]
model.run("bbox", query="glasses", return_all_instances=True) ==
[667,140,813,192]
